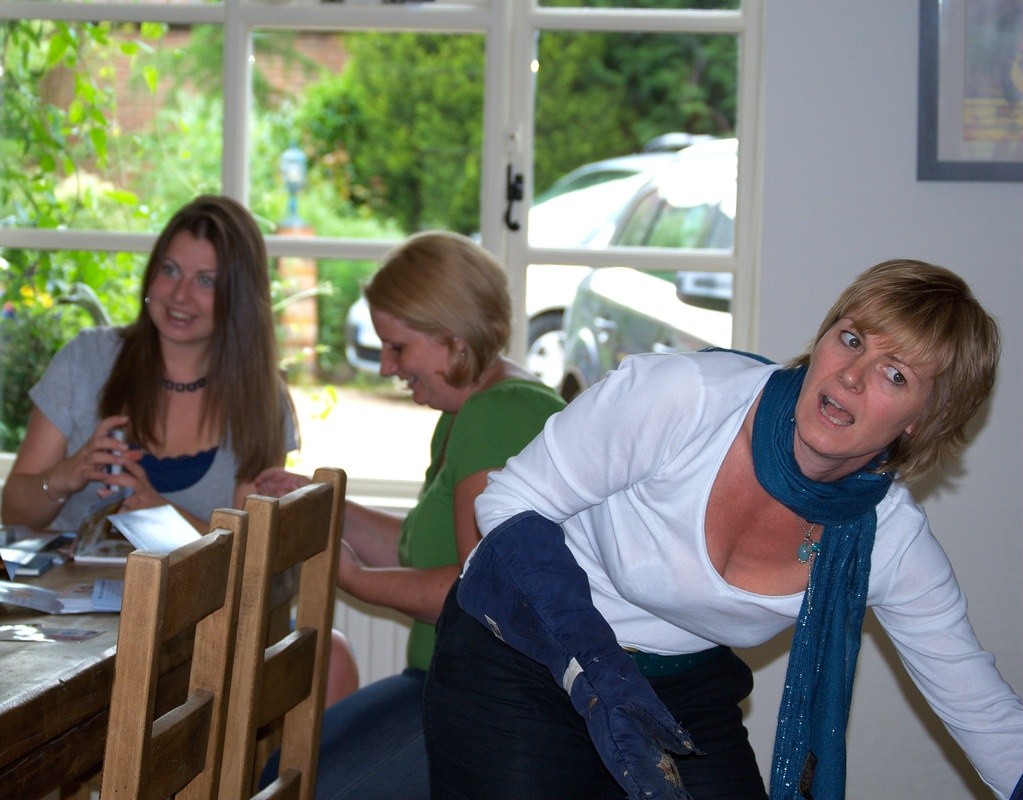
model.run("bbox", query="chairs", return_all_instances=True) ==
[218,467,348,800]
[99,507,249,800]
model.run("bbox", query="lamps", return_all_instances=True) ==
[277,148,308,227]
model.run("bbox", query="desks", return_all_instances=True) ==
[0,533,292,800]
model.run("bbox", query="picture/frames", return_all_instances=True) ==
[916,0,1023,181]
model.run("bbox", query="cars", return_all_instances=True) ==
[343,132,740,403]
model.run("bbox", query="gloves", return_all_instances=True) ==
[456,513,694,800]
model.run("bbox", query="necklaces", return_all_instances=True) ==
[793,513,821,627]
[159,376,206,392]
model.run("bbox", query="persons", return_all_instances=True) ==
[419,258,1023,800]
[241,233,567,800]
[1,194,361,711]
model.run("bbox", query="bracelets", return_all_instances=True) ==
[41,479,66,504]
